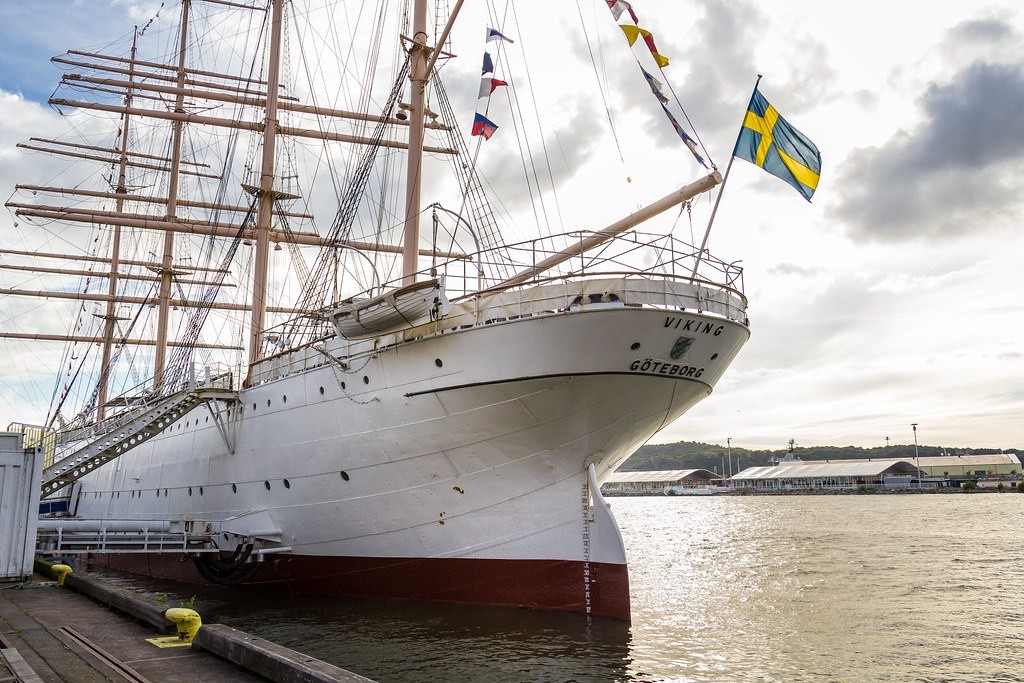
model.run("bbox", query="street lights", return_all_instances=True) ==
[910,423,922,487]
[726,437,732,477]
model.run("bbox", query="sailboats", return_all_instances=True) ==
[1,2,753,626]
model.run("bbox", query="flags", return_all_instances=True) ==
[471,27,514,141]
[734,88,823,203]
[605,0,670,70]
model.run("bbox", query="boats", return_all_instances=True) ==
[329,280,440,339]
